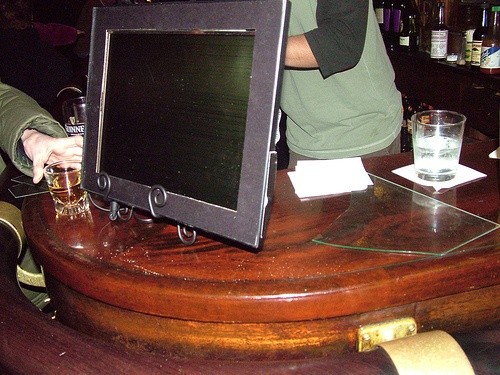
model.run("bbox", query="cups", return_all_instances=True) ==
[411,109,467,181]
[43,159,89,216]
[61,96,86,138]
[410,182,462,231]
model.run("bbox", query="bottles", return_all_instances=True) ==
[371,0,500,75]
[400,94,434,153]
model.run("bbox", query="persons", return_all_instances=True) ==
[0,81,84,319]
[280,0,403,169]
